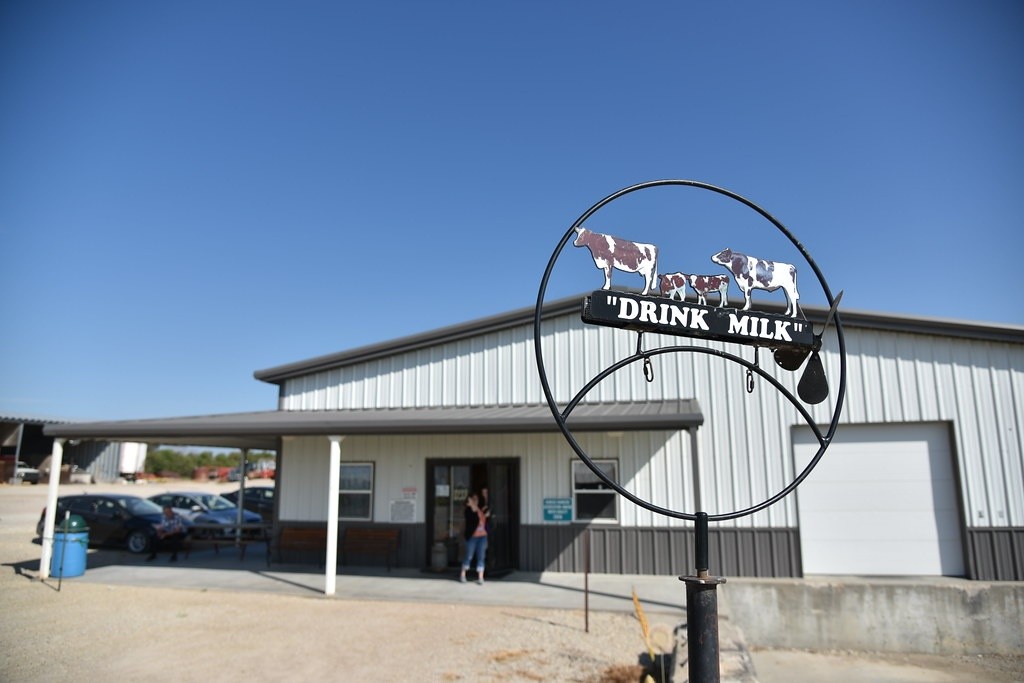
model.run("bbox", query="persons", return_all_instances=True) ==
[144,504,189,563]
[458,493,491,586]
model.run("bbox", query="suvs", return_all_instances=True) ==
[14,462,41,484]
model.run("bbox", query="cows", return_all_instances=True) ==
[711,247,801,317]
[658,272,686,302]
[686,273,730,308]
[573,226,658,296]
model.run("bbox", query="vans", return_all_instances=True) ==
[32,493,192,552]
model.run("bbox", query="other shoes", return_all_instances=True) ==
[477,578,484,584]
[460,575,467,583]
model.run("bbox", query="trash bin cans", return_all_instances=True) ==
[53,514,89,577]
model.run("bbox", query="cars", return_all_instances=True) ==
[222,487,273,517]
[149,494,263,539]
